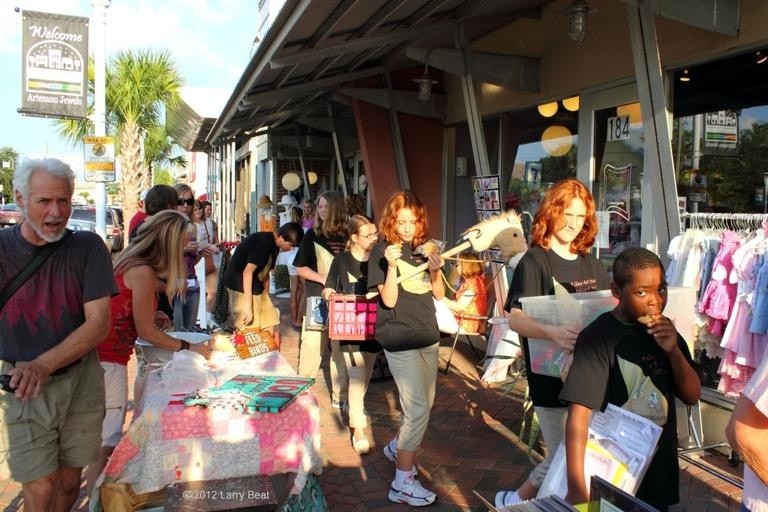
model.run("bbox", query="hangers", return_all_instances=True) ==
[671,210,767,250]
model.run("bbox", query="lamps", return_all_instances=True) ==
[306,171,318,186]
[560,1,596,46]
[534,93,644,160]
[281,157,300,193]
[410,43,459,108]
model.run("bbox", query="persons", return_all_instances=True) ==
[490,178,614,506]
[367,189,446,507]
[86,209,210,503]
[725,343,768,511]
[560,248,702,510]
[0,157,122,512]
[129,183,492,454]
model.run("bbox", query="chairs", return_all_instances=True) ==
[435,296,500,376]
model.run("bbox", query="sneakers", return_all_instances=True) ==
[351,429,369,457]
[331,398,349,411]
[494,490,516,507]
[382,438,418,479]
[388,478,438,507]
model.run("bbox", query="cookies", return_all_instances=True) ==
[637,315,652,325]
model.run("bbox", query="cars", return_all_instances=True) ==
[0,201,124,257]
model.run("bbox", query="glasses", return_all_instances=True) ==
[358,232,380,238]
[178,198,194,207]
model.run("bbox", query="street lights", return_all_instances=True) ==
[759,172,768,213]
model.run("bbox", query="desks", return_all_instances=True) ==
[85,334,331,511]
